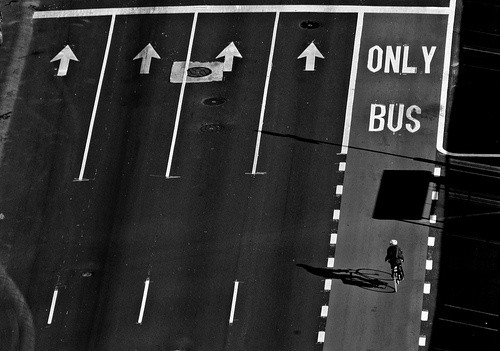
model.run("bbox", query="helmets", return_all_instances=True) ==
[390,239,398,245]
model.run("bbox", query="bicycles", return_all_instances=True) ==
[386,259,404,293]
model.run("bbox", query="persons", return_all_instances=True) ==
[385,239,405,281]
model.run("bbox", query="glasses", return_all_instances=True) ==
[390,243,392,245]
